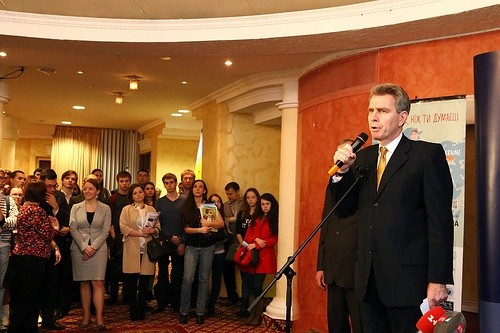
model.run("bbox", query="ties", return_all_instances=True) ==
[376,146,389,190]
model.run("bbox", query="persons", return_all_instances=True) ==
[179,179,224,323]
[330,81,453,333]
[315,139,363,333]
[0,168,195,333]
[206,182,278,316]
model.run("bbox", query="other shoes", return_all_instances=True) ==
[236,310,249,317]
[196,313,203,325]
[138,307,146,320]
[207,307,216,316]
[179,314,187,324]
[153,304,166,314]
[118,301,131,305]
[221,297,240,305]
[144,303,154,311]
[43,321,65,330]
[129,311,138,321]
[104,298,117,305]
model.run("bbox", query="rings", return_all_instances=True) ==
[439,301,444,304]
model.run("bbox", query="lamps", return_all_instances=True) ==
[112,91,126,104]
[124,74,144,91]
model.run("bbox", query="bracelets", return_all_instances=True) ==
[54,246,59,251]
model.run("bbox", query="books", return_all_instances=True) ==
[144,211,161,229]
[199,204,218,232]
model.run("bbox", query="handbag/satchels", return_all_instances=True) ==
[234,246,253,266]
[147,227,169,262]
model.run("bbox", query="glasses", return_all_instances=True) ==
[45,183,59,189]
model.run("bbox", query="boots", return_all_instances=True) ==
[242,295,255,324]
[251,298,264,325]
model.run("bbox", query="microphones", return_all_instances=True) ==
[328,132,369,176]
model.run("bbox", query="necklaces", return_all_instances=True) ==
[133,202,145,210]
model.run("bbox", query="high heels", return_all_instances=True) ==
[96,324,105,333]
[80,313,93,328]
